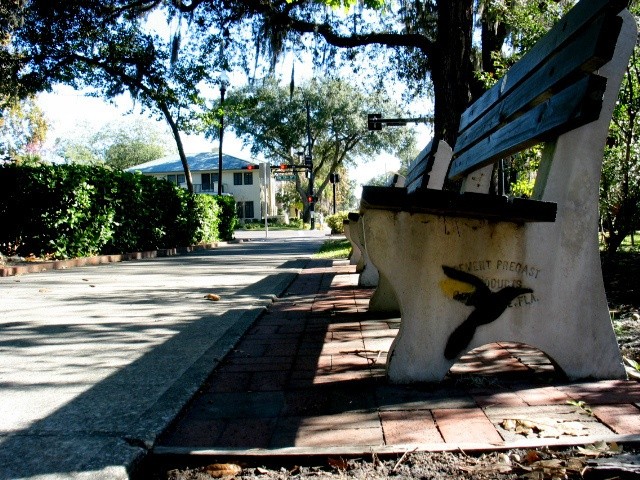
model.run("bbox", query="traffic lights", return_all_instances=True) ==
[247,165,252,170]
[308,197,312,202]
[280,164,287,169]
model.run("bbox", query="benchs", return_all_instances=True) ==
[342,173,406,266]
[361,0,637,390]
[349,136,454,316]
